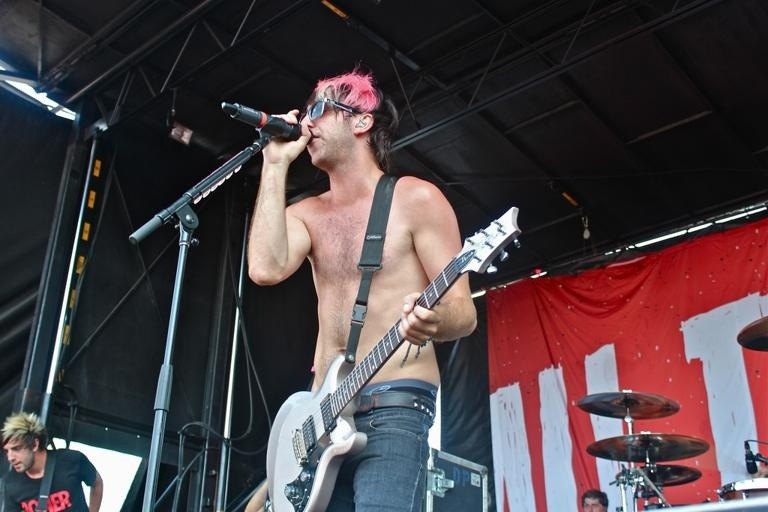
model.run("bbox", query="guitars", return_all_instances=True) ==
[266,206,521,510]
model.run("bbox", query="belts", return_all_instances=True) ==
[360,389,436,418]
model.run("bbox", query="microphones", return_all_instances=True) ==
[222,102,313,145]
[744,441,757,474]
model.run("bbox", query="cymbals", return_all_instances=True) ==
[615,464,701,486]
[577,392,680,418]
[586,432,710,462]
[738,317,767,350]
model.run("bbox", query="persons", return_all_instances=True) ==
[1,411,104,512]
[579,487,609,512]
[247,69,481,512]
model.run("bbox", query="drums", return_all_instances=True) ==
[716,478,768,499]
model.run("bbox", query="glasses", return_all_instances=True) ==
[298,97,362,125]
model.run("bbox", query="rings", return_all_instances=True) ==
[426,335,433,342]
[420,342,427,348]
